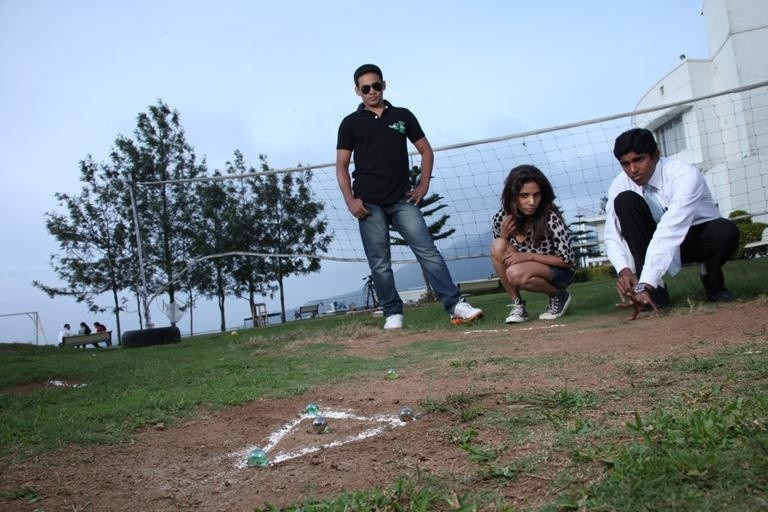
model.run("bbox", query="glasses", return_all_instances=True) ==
[361,82,382,95]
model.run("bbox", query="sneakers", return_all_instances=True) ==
[699,271,731,302]
[449,295,483,326]
[538,288,572,320]
[639,284,670,311]
[505,297,530,324]
[384,314,404,329]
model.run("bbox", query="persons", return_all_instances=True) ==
[77,322,92,348]
[602,130,741,319]
[336,63,484,331]
[59,323,79,348]
[490,165,580,323]
[91,322,106,347]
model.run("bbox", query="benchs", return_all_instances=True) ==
[742,227,767,260]
[456,278,502,297]
[293,304,320,320]
[58,330,112,349]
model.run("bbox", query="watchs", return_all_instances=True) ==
[632,284,650,294]
